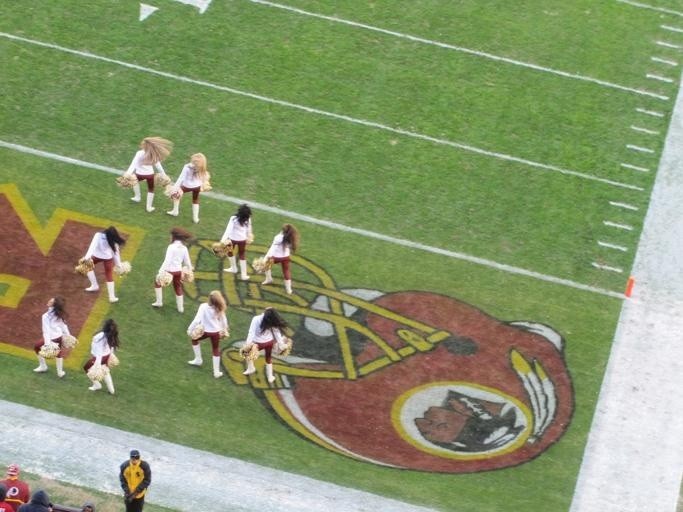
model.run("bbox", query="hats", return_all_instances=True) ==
[6,464,20,476]
[130,451,139,458]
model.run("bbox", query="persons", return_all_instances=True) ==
[167,151,210,225]
[260,223,295,296]
[149,228,193,313]
[15,489,50,511]
[240,306,284,383]
[121,136,168,213]
[184,290,228,379]
[218,203,254,281]
[30,294,67,378]
[82,318,118,394]
[82,225,125,303]
[0,463,28,511]
[0,482,15,512]
[117,448,151,511]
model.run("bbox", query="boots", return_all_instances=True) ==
[105,280,119,303]
[55,357,65,378]
[223,256,292,294]
[33,354,48,373]
[242,361,256,375]
[176,294,185,314]
[188,343,203,366]
[212,355,223,379]
[130,183,200,224]
[84,270,99,291]
[88,373,115,395]
[265,362,276,383]
[152,287,163,308]
